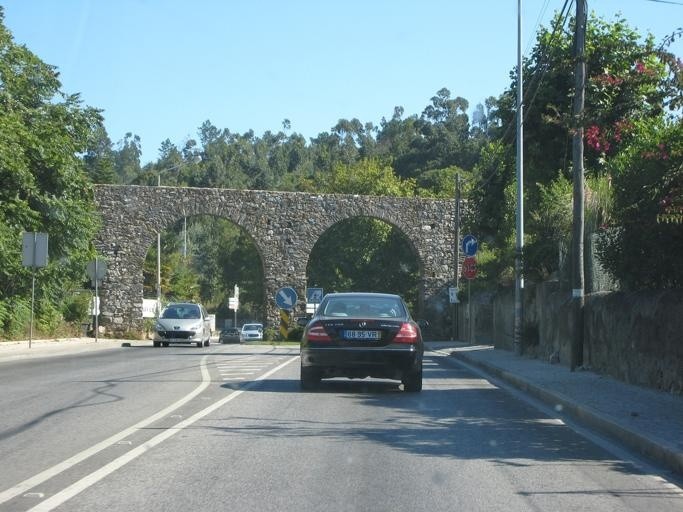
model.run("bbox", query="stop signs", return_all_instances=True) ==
[462,258,477,279]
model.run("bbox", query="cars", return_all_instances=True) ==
[152,299,213,350]
[218,323,264,343]
[299,292,431,394]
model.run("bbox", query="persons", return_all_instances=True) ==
[330,301,347,313]
[360,304,370,313]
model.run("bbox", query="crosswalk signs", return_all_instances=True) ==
[308,288,322,304]
